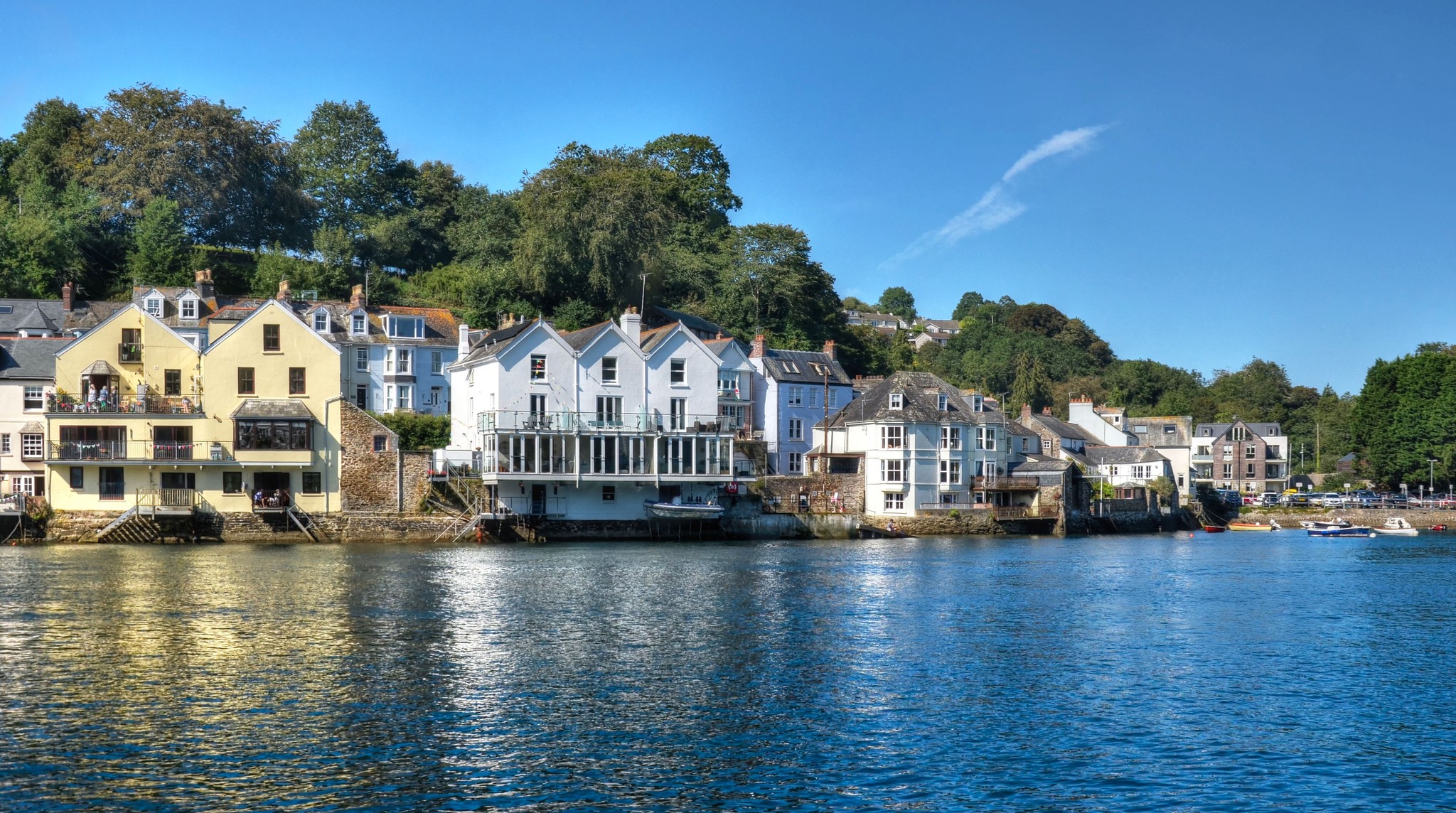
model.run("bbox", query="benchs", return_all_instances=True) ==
[172,402,192,411]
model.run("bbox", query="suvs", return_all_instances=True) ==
[1351,490,1382,508]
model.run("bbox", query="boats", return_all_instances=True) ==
[1204,525,1227,533]
[1428,524,1449,531]
[642,498,726,519]
[1228,519,1283,531]
[1372,516,1420,536]
[1298,517,1372,537]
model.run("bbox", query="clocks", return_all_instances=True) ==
[499,442,510,454]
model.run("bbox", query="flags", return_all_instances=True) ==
[0,494,18,503]
[154,443,202,450]
[735,376,740,400]
[71,442,100,449]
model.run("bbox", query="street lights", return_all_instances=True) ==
[1426,459,1438,496]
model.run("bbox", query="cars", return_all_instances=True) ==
[1215,488,1257,505]
[1256,490,1359,509]
[1378,489,1456,510]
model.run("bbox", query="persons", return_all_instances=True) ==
[887,519,897,532]
[122,343,128,360]
[88,384,97,402]
[255,489,281,508]
[182,396,191,412]
[110,386,117,405]
[1398,519,1404,529]
[99,386,108,407]
[832,489,843,513]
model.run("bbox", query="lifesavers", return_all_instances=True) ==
[763,505,770,512]
[728,483,737,490]
[1390,519,1394,523]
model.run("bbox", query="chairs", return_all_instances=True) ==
[523,415,536,430]
[538,416,554,430]
[146,398,158,411]
[159,398,171,411]
[686,420,721,432]
[572,419,640,431]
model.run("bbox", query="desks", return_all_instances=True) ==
[74,404,86,413]
[494,465,505,472]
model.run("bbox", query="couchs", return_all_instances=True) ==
[634,461,649,474]
[529,460,573,473]
[580,460,590,473]
